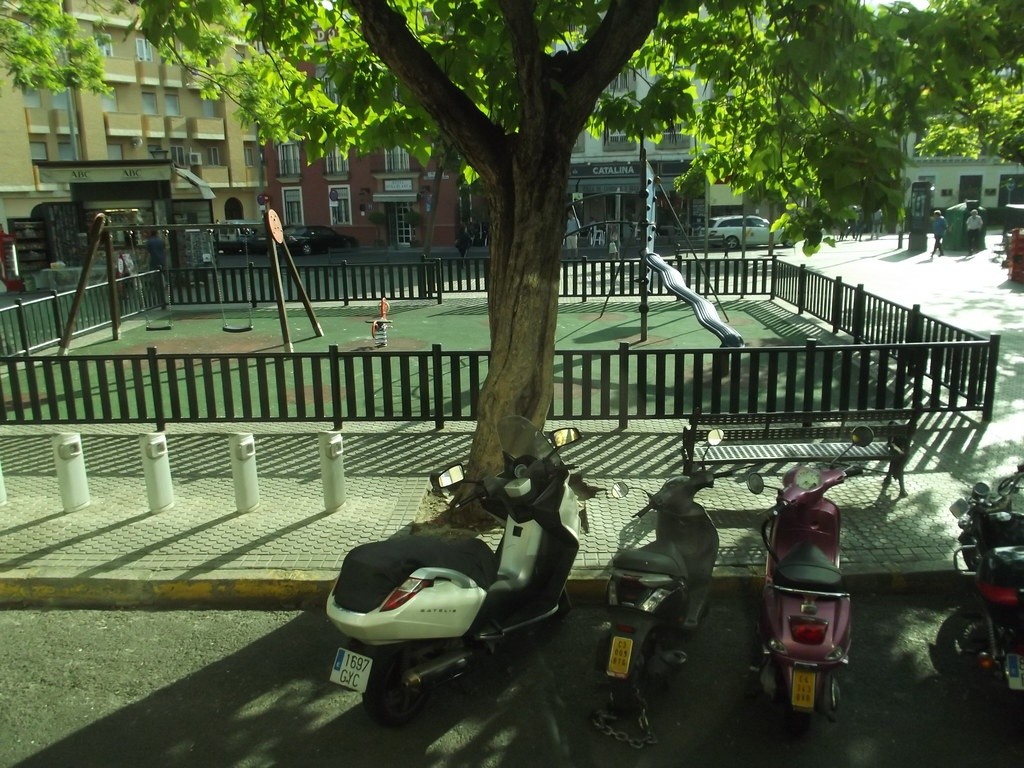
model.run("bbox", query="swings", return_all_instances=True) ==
[205,228,254,333]
[128,230,172,331]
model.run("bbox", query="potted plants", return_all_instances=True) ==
[365,213,386,250]
[405,210,422,248]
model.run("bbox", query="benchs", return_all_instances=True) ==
[680,401,924,497]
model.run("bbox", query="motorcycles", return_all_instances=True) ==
[937,462,1023,751]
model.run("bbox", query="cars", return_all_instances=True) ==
[273,225,357,257]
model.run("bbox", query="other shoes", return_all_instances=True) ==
[968,252,973,256]
[976,249,979,252]
[931,254,933,258]
[938,252,944,257]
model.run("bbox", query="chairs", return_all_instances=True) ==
[589,221,605,247]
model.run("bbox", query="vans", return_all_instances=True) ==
[218,219,259,243]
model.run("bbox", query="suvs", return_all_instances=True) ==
[699,216,796,251]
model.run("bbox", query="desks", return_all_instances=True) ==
[34,267,103,290]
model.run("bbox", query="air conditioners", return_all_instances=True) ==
[185,152,202,166]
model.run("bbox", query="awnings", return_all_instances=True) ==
[175,168,217,199]
[567,177,675,192]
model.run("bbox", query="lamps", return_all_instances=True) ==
[129,137,143,150]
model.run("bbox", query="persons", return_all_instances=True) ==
[966,209,982,256]
[143,231,166,271]
[838,205,865,242]
[870,209,882,240]
[565,212,578,260]
[930,210,949,258]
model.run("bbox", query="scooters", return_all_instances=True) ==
[746,424,874,737]
[597,430,733,714]
[326,414,580,727]
